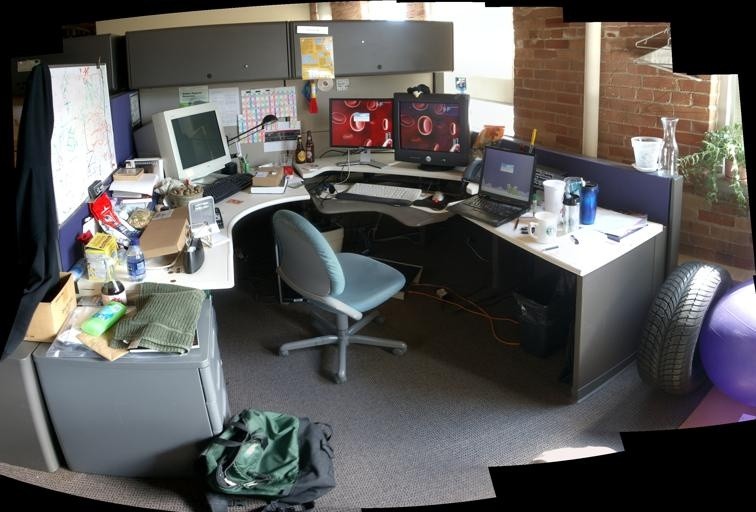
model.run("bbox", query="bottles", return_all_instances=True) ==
[581,183,598,225]
[100,261,127,306]
[306,130,314,163]
[127,238,149,282]
[563,193,580,233]
[295,135,305,163]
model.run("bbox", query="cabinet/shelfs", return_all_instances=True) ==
[61,33,124,92]
[11,54,63,98]
[35,296,228,478]
[290,20,454,80]
[125,21,292,89]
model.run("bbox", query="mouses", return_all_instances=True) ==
[432,190,443,204]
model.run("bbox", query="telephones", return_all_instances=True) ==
[462,157,483,181]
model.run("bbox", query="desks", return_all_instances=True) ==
[32,151,669,479]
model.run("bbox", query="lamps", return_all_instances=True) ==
[227,113,279,145]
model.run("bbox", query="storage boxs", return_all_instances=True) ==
[23,271,79,343]
[139,206,191,263]
[253,166,285,187]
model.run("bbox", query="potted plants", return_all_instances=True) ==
[677,119,750,214]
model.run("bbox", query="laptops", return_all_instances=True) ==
[447,145,536,226]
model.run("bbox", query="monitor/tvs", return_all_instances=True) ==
[330,98,394,169]
[394,93,468,171]
[134,103,231,184]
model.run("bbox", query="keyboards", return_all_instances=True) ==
[203,173,253,203]
[345,182,422,207]
[337,194,343,195]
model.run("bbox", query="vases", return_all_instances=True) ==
[629,135,664,174]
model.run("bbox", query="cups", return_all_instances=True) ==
[631,134,663,167]
[526,210,560,246]
[542,178,566,210]
[578,185,599,225]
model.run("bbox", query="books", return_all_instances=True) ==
[130,322,200,353]
[109,157,166,211]
[250,166,288,194]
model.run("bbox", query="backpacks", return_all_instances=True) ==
[198,408,335,512]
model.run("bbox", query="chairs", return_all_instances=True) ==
[272,208,409,385]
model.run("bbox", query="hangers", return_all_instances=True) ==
[648,63,703,83]
[635,27,671,50]
[633,37,672,65]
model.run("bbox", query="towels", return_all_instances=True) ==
[108,282,207,357]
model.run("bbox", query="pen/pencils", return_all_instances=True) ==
[570,235,579,244]
[542,246,558,251]
[513,216,520,231]
